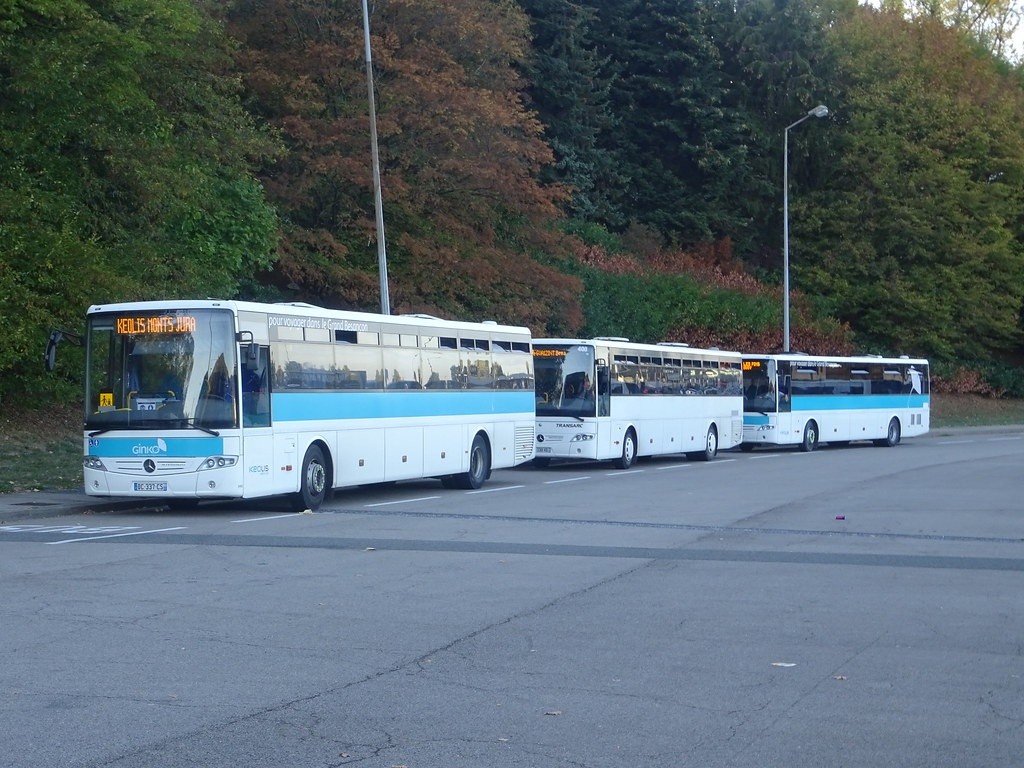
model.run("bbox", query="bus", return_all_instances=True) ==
[742,350,930,452]
[45,299,537,512]
[530,336,744,469]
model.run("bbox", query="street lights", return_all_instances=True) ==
[784,103,829,356]
